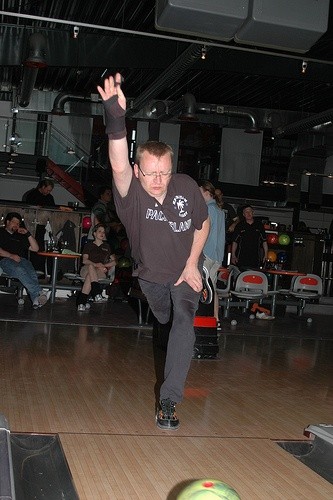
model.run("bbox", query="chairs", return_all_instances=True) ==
[291,273,322,317]
[67,266,115,304]
[0,271,43,305]
[215,264,268,319]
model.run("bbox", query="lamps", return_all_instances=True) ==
[202,45,207,60]
[73,25,80,39]
[301,60,308,73]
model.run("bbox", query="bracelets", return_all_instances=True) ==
[24,231,32,238]
[104,264,106,268]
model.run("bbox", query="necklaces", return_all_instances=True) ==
[94,240,102,246]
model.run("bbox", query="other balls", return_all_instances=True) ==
[85,303,90,309]
[230,320,238,325]
[267,234,279,244]
[267,251,277,262]
[82,217,92,228]
[278,235,290,245]
[249,314,255,319]
[307,317,312,323]
[117,257,131,268]
[18,299,24,304]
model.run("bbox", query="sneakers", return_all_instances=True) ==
[155,401,180,432]
[199,267,213,304]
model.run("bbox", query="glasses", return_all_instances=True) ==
[137,164,173,181]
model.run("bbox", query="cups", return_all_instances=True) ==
[74,201,79,211]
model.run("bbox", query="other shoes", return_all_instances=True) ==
[33,291,52,309]
[78,303,86,312]
[95,294,102,302]
[256,311,274,321]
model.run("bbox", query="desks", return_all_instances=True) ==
[40,253,80,305]
[266,270,306,316]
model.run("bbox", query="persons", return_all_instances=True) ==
[75,225,116,313]
[94,185,270,317]
[0,211,52,310]
[97,71,209,431]
[20,177,74,212]
